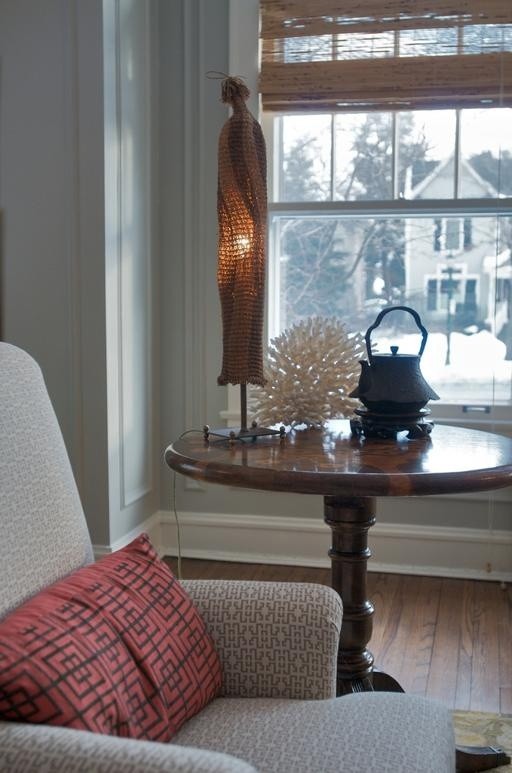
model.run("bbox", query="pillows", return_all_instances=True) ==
[0,533,224,744]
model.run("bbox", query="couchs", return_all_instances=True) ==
[0,339,459,772]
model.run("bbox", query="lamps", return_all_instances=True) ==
[202,69,285,447]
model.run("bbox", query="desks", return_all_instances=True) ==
[163,415,511,771]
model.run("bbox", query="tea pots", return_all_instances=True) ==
[349,305,443,416]
